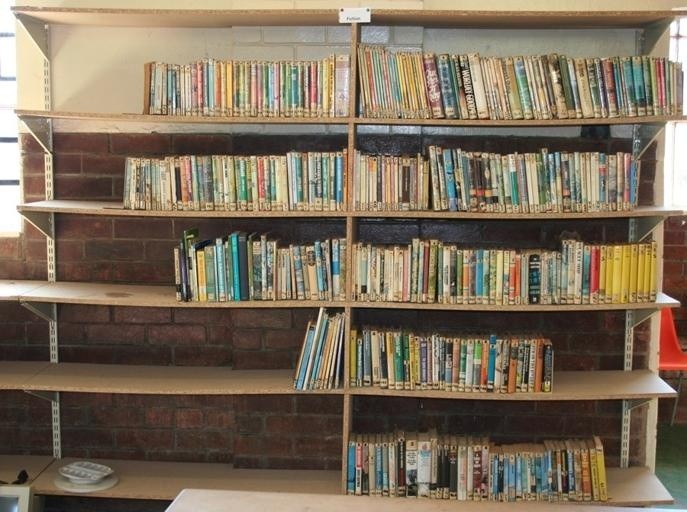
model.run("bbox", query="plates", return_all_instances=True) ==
[54,476,119,494]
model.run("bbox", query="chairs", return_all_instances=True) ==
[658,306,687,425]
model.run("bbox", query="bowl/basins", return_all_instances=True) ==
[58,464,111,484]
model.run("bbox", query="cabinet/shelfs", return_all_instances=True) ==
[0,5,686,512]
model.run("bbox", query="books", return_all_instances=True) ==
[291,305,345,392]
[347,427,610,502]
[350,323,557,394]
[352,142,636,213]
[350,231,658,302]
[356,41,686,118]
[121,147,348,213]
[171,223,346,303]
[141,51,350,119]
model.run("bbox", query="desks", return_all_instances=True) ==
[163,486,687,512]
